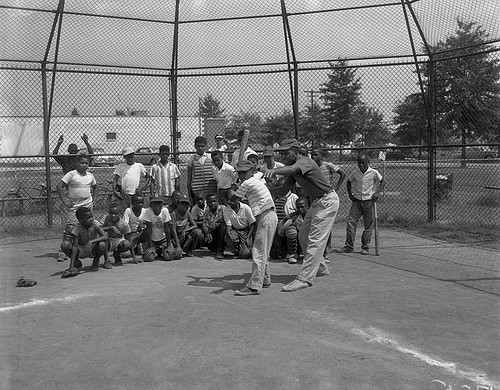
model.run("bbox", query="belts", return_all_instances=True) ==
[318,188,333,198]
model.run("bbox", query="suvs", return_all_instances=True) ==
[457,146,496,159]
[378,142,406,161]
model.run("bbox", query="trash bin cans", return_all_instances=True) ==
[434,171,454,205]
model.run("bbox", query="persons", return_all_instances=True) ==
[259,148,284,173]
[274,177,299,264]
[296,196,309,217]
[223,190,256,258]
[149,145,181,205]
[202,193,226,259]
[261,138,339,292]
[191,191,206,227]
[99,203,130,269]
[298,144,308,157]
[112,147,149,207]
[229,131,258,169]
[341,154,385,255]
[187,135,213,204]
[122,194,146,264]
[53,133,94,176]
[143,194,175,262]
[247,153,259,170]
[166,190,183,213]
[61,207,108,278]
[170,194,202,257]
[264,174,278,200]
[57,154,97,262]
[227,160,278,296]
[208,133,227,152]
[310,144,346,190]
[211,150,236,204]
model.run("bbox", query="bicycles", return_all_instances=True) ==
[28,190,68,215]
[2,173,68,217]
[92,177,113,208]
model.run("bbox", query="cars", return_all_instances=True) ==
[77,148,115,166]
[122,146,172,165]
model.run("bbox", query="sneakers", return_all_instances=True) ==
[281,279,309,292]
[263,284,272,288]
[235,286,259,296]
[360,249,369,255]
[289,258,297,263]
[337,246,353,253]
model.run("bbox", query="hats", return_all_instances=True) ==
[122,147,135,156]
[274,139,300,151]
[178,194,189,202]
[233,160,254,173]
[149,193,163,202]
[263,145,274,156]
[214,133,224,139]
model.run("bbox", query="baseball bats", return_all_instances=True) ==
[373,201,380,255]
[228,129,250,205]
